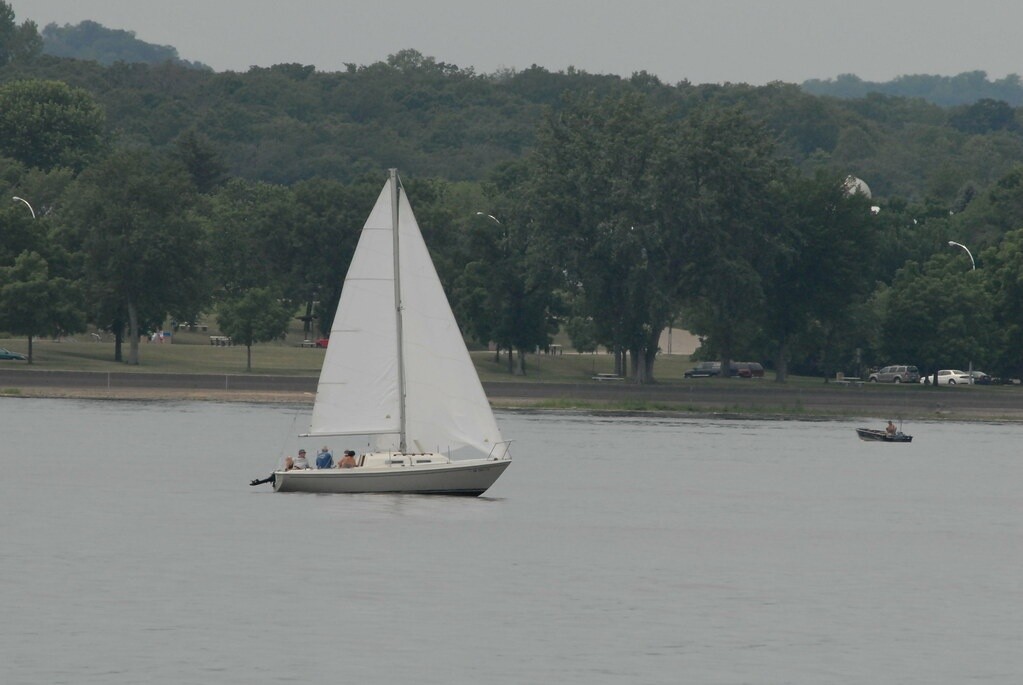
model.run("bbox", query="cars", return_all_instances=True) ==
[729,361,764,379]
[919,370,975,385]
[966,370,991,385]
[0,348,29,360]
[684,361,722,379]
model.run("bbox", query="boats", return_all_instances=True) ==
[855,427,913,443]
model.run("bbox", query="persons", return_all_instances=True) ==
[886,421,895,435]
[338,450,356,468]
[287,450,309,470]
[316,446,334,469]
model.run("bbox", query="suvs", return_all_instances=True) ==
[869,365,920,384]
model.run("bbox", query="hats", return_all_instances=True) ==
[321,445,329,450]
[298,449,306,454]
[343,450,348,455]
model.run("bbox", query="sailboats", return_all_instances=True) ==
[249,167,513,498]
